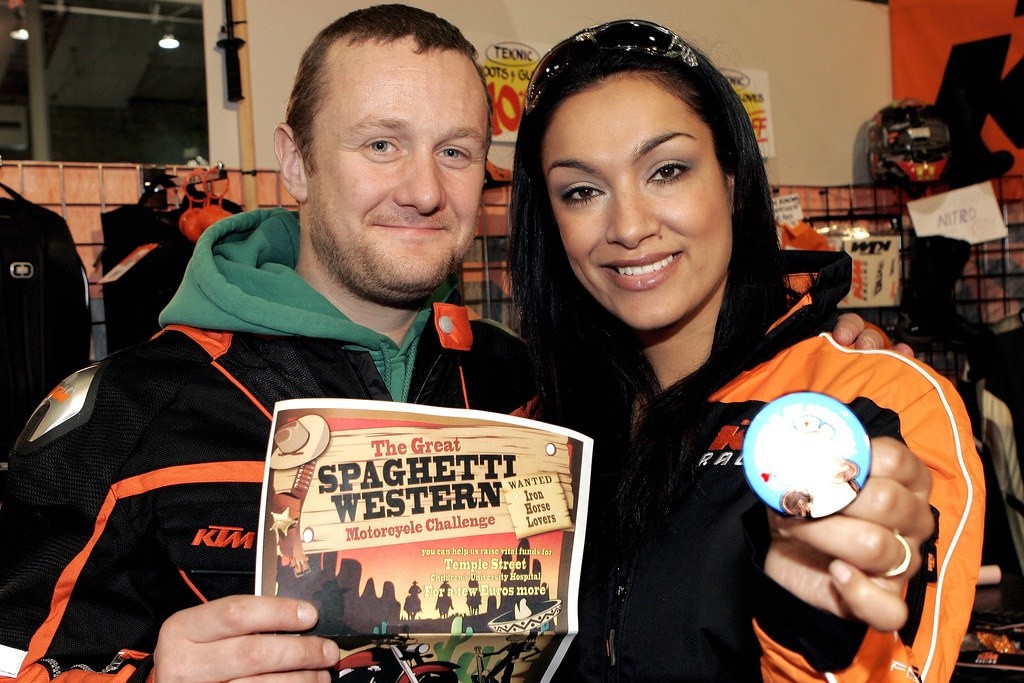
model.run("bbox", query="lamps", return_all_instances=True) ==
[156,25,182,51]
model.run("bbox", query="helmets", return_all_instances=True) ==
[867,96,951,186]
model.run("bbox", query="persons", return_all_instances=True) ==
[0,4,914,683]
[504,18,988,683]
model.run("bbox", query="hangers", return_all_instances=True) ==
[0,170,39,213]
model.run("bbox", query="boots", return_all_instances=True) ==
[896,236,994,351]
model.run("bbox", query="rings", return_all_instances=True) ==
[885,534,911,577]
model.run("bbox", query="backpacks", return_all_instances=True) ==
[0,185,91,445]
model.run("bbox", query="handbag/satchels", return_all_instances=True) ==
[91,178,244,355]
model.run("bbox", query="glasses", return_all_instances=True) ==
[525,21,698,117]
